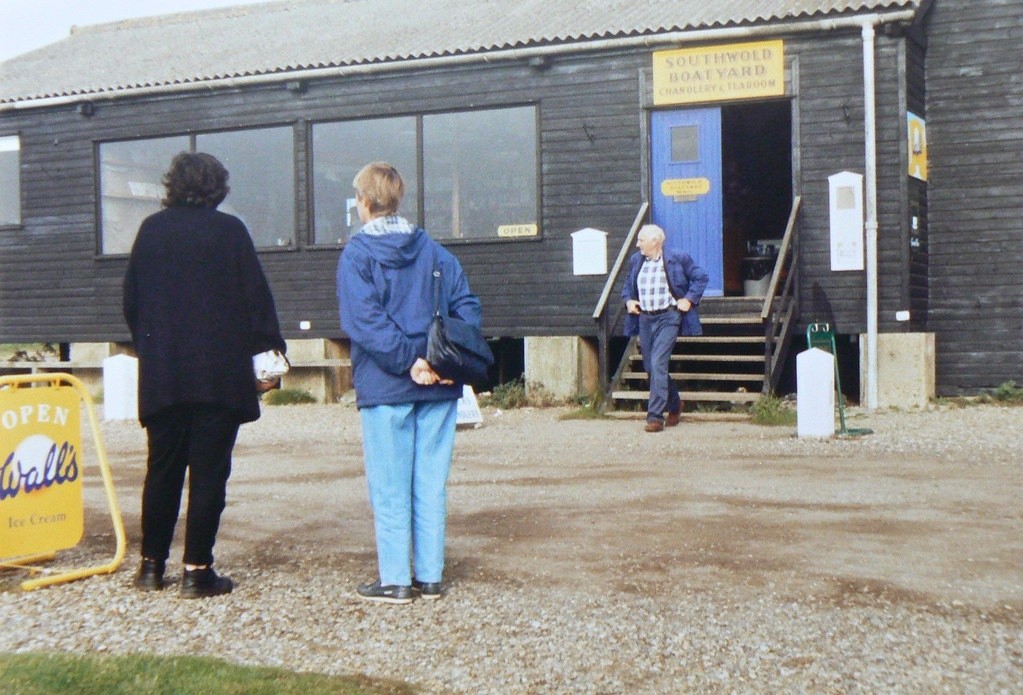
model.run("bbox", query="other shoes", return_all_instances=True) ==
[666,400,685,427]
[644,420,664,433]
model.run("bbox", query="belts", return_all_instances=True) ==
[639,306,678,318]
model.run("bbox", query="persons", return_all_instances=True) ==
[122,151,287,600]
[621,225,708,432]
[337,161,484,603]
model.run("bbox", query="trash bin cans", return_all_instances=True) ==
[739,255,773,297]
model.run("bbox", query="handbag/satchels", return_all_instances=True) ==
[425,242,496,395]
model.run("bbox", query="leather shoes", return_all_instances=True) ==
[356,580,413,605]
[410,575,442,601]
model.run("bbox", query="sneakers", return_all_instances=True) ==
[180,566,233,600]
[134,559,166,592]
